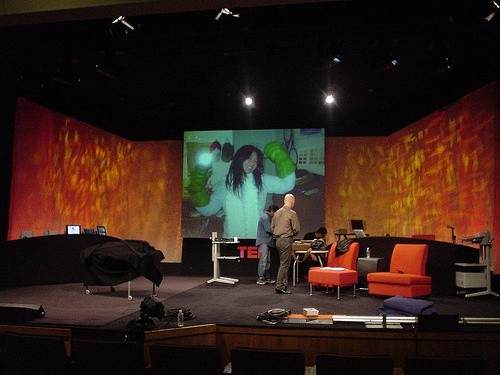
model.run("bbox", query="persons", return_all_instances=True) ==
[303,227,327,280]
[191,140,296,239]
[256,206,278,285]
[270,193,300,294]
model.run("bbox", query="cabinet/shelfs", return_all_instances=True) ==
[206,232,239,287]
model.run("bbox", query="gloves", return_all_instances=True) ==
[263,141,297,179]
[188,165,213,209]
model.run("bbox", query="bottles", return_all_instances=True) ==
[178,310,184,326]
[366,248,370,258]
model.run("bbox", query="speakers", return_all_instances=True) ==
[0,302,46,323]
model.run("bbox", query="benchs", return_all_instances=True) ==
[367,244,432,299]
[308,242,359,299]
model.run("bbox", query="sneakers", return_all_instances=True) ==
[257,277,276,285]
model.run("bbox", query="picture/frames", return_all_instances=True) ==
[84,229,95,233]
[66,225,81,234]
[97,226,107,236]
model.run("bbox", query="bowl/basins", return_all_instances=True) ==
[339,228,346,234]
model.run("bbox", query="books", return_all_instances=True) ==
[303,307,319,317]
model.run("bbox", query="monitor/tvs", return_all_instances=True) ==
[66,224,80,234]
[96,225,107,235]
[354,230,367,238]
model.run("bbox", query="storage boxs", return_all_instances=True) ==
[455,263,487,287]
[292,242,311,251]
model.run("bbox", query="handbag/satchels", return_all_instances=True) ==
[140,297,166,322]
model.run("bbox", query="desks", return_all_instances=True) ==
[293,251,329,286]
[335,234,369,241]
[357,257,385,291]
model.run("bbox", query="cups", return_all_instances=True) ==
[234,237,238,242]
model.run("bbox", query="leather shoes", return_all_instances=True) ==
[275,286,291,294]
